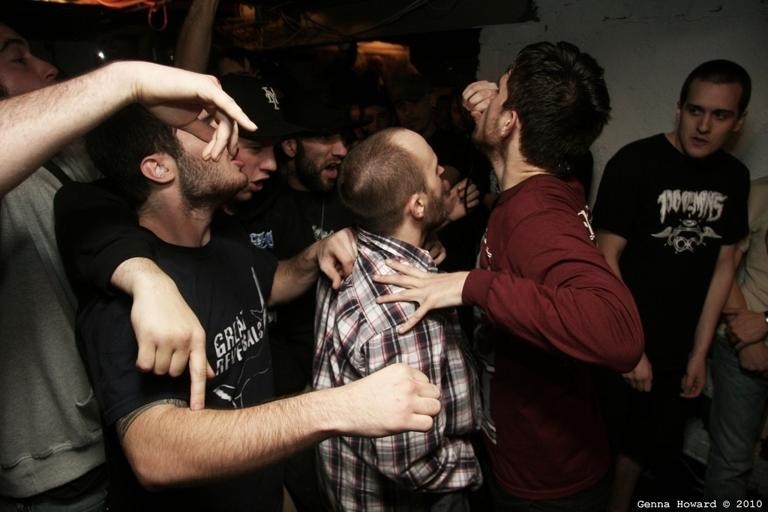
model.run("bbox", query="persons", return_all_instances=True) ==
[0,15,766,512]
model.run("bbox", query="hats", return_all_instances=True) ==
[284,81,372,137]
[217,70,290,146]
[391,74,431,102]
